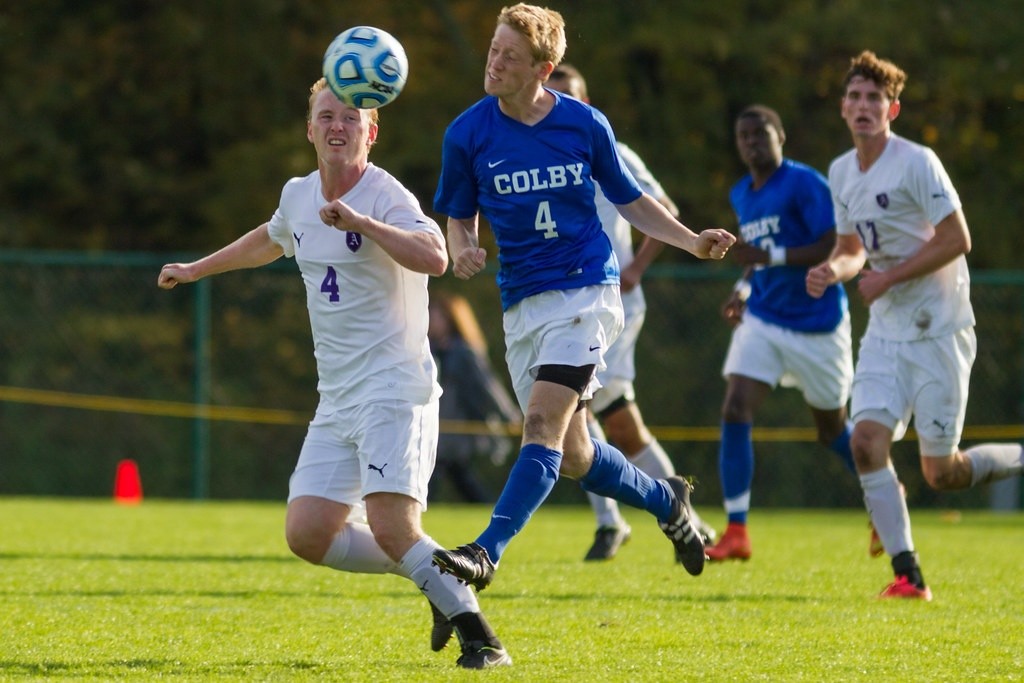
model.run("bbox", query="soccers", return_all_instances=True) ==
[323,25,409,111]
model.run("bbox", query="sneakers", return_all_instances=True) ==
[878,576,933,603]
[672,524,713,563]
[427,594,456,653]
[658,477,705,576]
[870,523,882,559]
[584,523,632,561]
[702,525,752,559]
[430,543,499,593]
[455,638,510,669]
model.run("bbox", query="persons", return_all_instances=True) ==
[806,50,1023,601]
[433,2,737,592]
[547,65,716,562]
[705,109,909,562]
[157,80,513,671]
